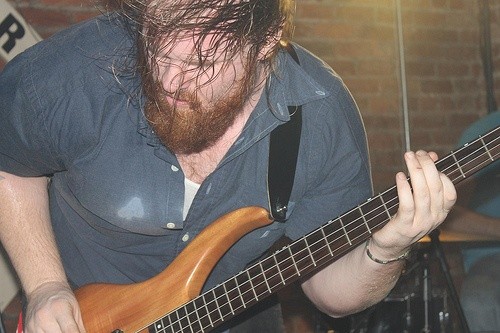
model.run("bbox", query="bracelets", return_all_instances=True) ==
[366,239,411,264]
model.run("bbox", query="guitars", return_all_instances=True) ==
[9,119,500,333]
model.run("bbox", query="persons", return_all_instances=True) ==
[1,1,459,333]
